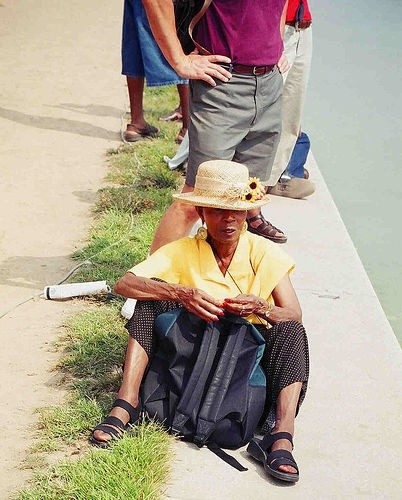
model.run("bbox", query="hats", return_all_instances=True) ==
[171,159,272,210]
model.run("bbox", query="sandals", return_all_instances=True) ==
[125,122,159,142]
[90,397,145,447]
[246,426,300,482]
[245,211,288,243]
[177,129,187,144]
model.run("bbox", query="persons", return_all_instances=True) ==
[121,0,189,142]
[142,1,290,256]
[90,160,309,480]
[248,0,313,240]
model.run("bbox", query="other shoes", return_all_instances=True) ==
[266,170,317,199]
[178,160,188,176]
[158,111,184,122]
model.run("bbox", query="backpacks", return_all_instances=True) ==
[137,306,269,451]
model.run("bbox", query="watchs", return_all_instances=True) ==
[264,302,271,318]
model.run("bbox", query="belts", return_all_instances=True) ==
[216,61,276,76]
[285,21,311,31]
